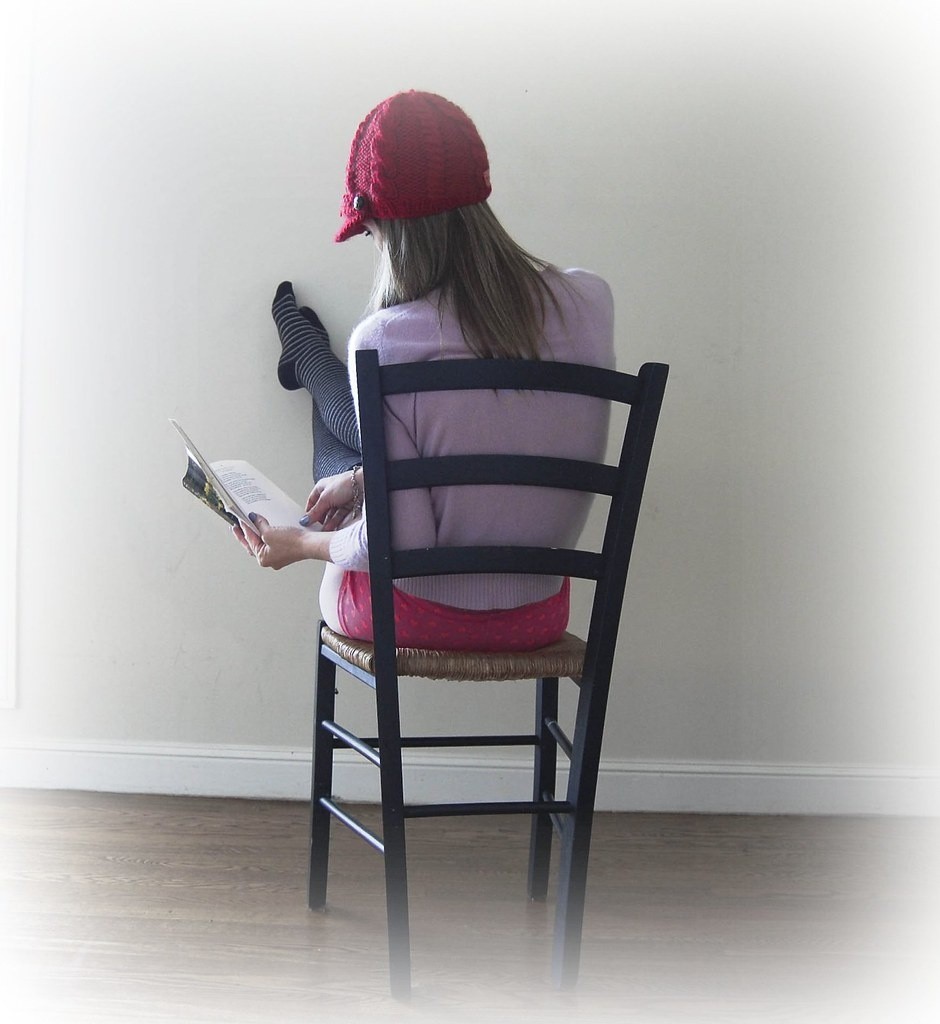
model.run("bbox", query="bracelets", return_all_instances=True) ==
[349,466,365,518]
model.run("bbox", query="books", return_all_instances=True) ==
[171,418,326,543]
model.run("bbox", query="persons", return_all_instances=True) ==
[272,89,614,651]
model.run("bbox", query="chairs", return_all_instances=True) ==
[302,348,669,1003]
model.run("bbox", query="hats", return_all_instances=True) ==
[334,89,491,243]
